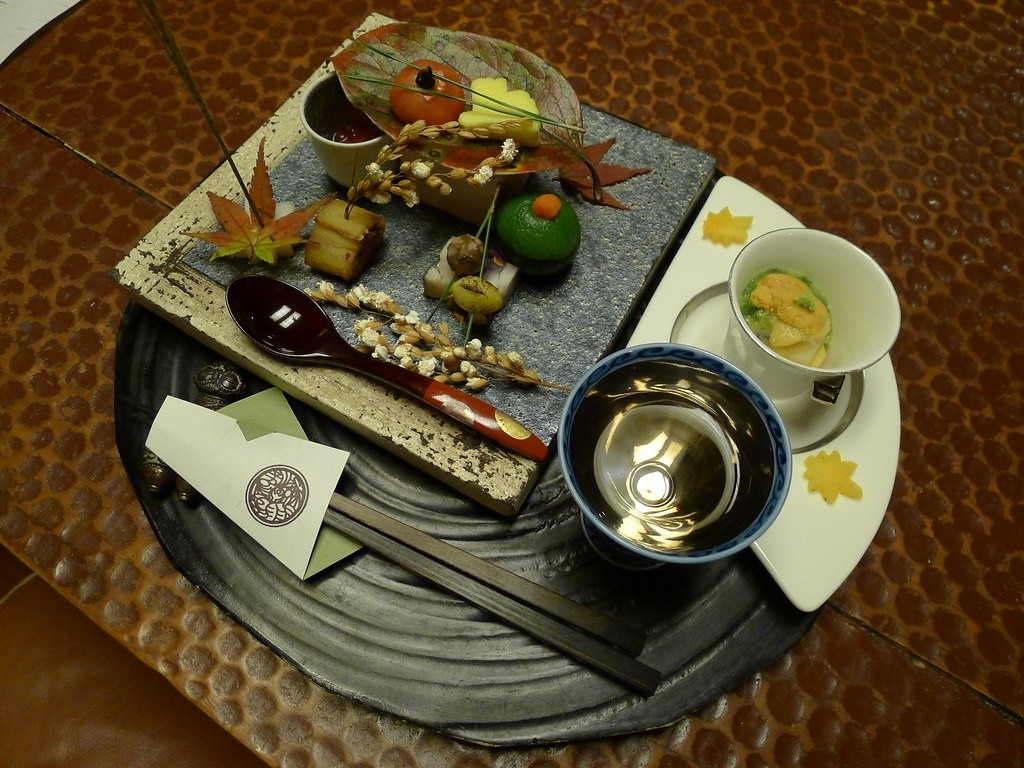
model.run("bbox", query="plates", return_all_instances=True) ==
[625,177,902,612]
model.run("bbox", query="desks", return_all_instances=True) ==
[0,0,1024,768]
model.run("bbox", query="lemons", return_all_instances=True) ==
[494,188,581,278]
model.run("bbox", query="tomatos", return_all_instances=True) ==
[390,59,465,131]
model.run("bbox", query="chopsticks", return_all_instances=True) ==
[322,492,663,699]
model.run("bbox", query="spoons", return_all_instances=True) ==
[224,274,549,462]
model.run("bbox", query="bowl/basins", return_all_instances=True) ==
[557,342,789,571]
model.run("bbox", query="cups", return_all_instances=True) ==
[300,71,396,191]
[718,228,902,398]
